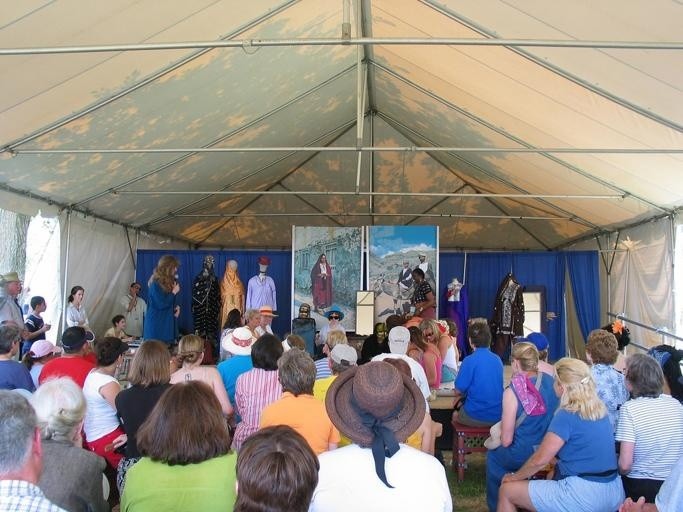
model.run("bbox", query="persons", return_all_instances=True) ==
[319,306,347,349]
[0,387,66,512]
[484,341,559,512]
[38,326,96,389]
[120,382,236,512]
[618,454,683,512]
[32,375,106,512]
[415,251,436,294]
[309,361,452,511]
[169,336,233,415]
[0,272,23,361]
[217,327,257,425]
[192,255,220,359]
[23,341,61,386]
[104,315,134,343]
[113,339,175,502]
[220,260,245,334]
[585,329,630,440]
[259,348,341,456]
[526,332,554,377]
[246,257,277,313]
[255,307,273,335]
[361,323,390,366]
[281,335,305,351]
[408,326,441,402]
[67,286,90,331]
[312,345,357,448]
[23,296,50,354]
[383,359,442,457]
[220,309,243,363]
[371,325,431,413]
[497,357,625,512]
[235,424,320,511]
[143,256,180,353]
[396,261,412,288]
[314,331,348,381]
[245,310,261,339]
[121,282,146,341]
[0,321,36,394]
[230,334,283,451]
[411,270,436,321]
[615,353,683,503]
[452,317,502,454]
[82,337,128,512]
[418,319,457,385]
[311,254,332,313]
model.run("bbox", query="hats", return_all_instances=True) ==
[325,360,426,451]
[259,306,280,317]
[389,326,410,354]
[29,340,62,358]
[222,327,257,355]
[257,256,271,265]
[282,339,292,353]
[323,305,344,321]
[484,421,502,449]
[0,272,23,283]
[62,330,94,349]
[331,344,358,365]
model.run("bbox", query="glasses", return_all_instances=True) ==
[18,337,21,342]
[424,332,434,337]
[131,285,141,291]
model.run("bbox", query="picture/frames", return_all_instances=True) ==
[291,224,363,332]
[366,224,439,331]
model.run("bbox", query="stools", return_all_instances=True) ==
[451,420,490,484]
